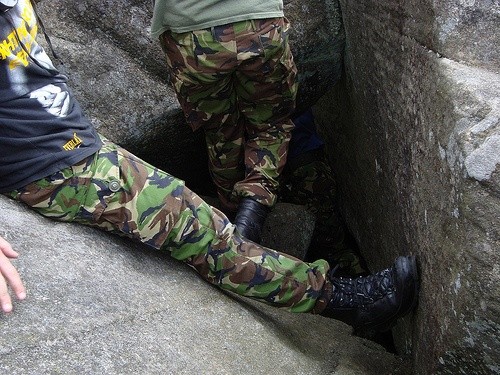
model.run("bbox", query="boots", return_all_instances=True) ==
[234,197,271,245]
[318,254,421,332]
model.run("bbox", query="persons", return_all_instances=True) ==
[0,0,419,335]
[148,0,298,242]
